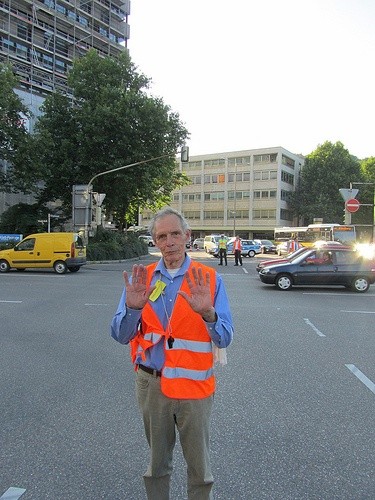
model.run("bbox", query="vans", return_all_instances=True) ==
[0,232,87,275]
[204,235,228,254]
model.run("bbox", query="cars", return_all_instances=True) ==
[259,249,375,293]
[275,242,304,256]
[193,238,205,249]
[311,240,343,248]
[253,240,276,254]
[255,244,354,272]
[210,240,261,258]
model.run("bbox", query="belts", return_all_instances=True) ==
[137,364,162,378]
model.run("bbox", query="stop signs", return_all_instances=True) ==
[345,199,360,212]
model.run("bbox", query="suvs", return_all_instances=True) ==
[138,236,155,247]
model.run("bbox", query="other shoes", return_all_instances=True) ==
[218,264,223,265]
[240,264,243,266]
[234,264,238,266]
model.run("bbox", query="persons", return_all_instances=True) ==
[319,252,333,264]
[231,235,243,266]
[217,234,228,266]
[110,206,235,500]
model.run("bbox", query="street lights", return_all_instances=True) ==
[48,214,60,233]
